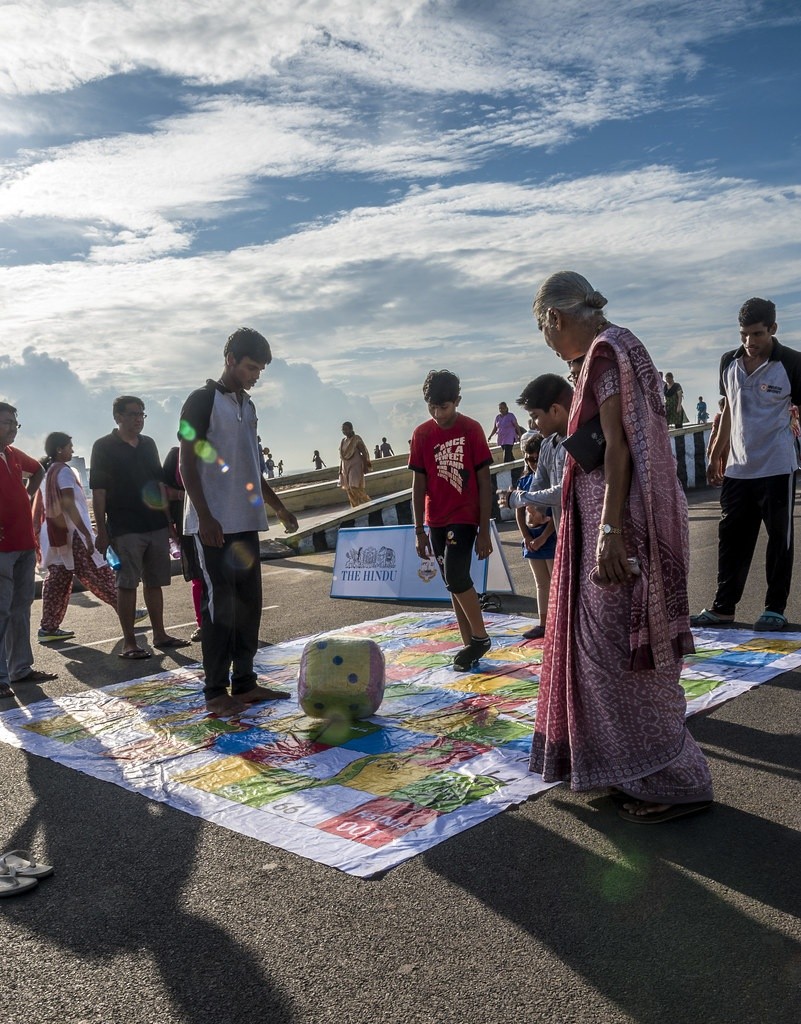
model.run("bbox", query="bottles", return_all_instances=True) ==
[170,538,180,560]
[105,545,121,570]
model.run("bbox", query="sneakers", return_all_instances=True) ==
[37,628,74,642]
[133,608,149,625]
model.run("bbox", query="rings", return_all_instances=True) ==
[90,550,93,552]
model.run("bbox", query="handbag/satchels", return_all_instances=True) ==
[561,412,606,474]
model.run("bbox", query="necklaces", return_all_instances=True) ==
[591,320,611,346]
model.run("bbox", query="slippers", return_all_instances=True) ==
[617,798,712,823]
[0,866,39,897]
[0,848,55,878]
[689,608,734,626]
[153,637,192,647]
[11,670,57,681]
[753,610,788,631]
[0,681,14,698]
[118,649,151,659]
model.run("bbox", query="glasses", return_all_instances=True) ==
[567,374,577,383]
[119,411,147,419]
[525,456,538,463]
[1,422,21,429]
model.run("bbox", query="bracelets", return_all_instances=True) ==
[414,525,425,535]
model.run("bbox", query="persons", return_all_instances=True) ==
[0,402,59,695]
[407,370,494,670]
[161,429,221,641]
[374,437,395,460]
[488,401,521,463]
[531,270,716,824]
[660,373,709,431]
[339,422,375,507]
[176,328,299,717]
[88,395,192,660]
[30,431,150,642]
[689,298,800,633]
[706,397,730,490]
[496,373,575,640]
[261,450,328,477]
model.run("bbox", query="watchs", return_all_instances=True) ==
[598,523,624,536]
